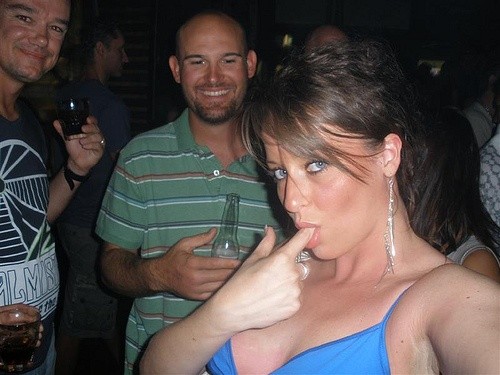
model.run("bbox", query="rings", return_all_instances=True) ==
[100,137,105,144]
[299,261,311,280]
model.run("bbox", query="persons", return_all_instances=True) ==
[0,0,500,375]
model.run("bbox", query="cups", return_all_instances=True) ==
[56,93,92,141]
[0,304,41,374]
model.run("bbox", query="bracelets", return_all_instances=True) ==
[63,160,88,191]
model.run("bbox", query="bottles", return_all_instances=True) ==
[211,193,240,259]
